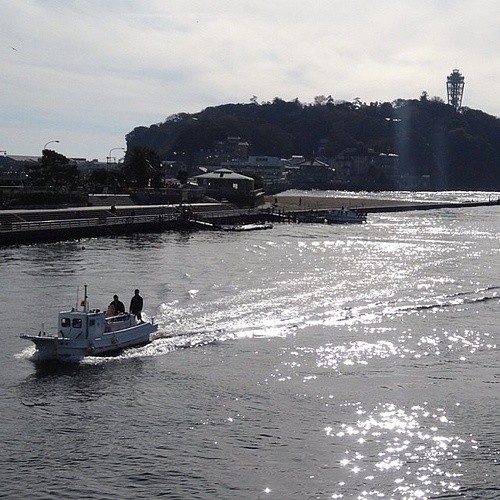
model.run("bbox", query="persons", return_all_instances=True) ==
[129,289,143,323]
[174,203,199,219]
[110,204,116,214]
[25,179,196,196]
[131,208,135,223]
[109,295,125,316]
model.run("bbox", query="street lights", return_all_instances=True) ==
[109,148,124,184]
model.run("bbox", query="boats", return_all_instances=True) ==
[324,206,368,224]
[223,223,273,231]
[18,283,158,363]
